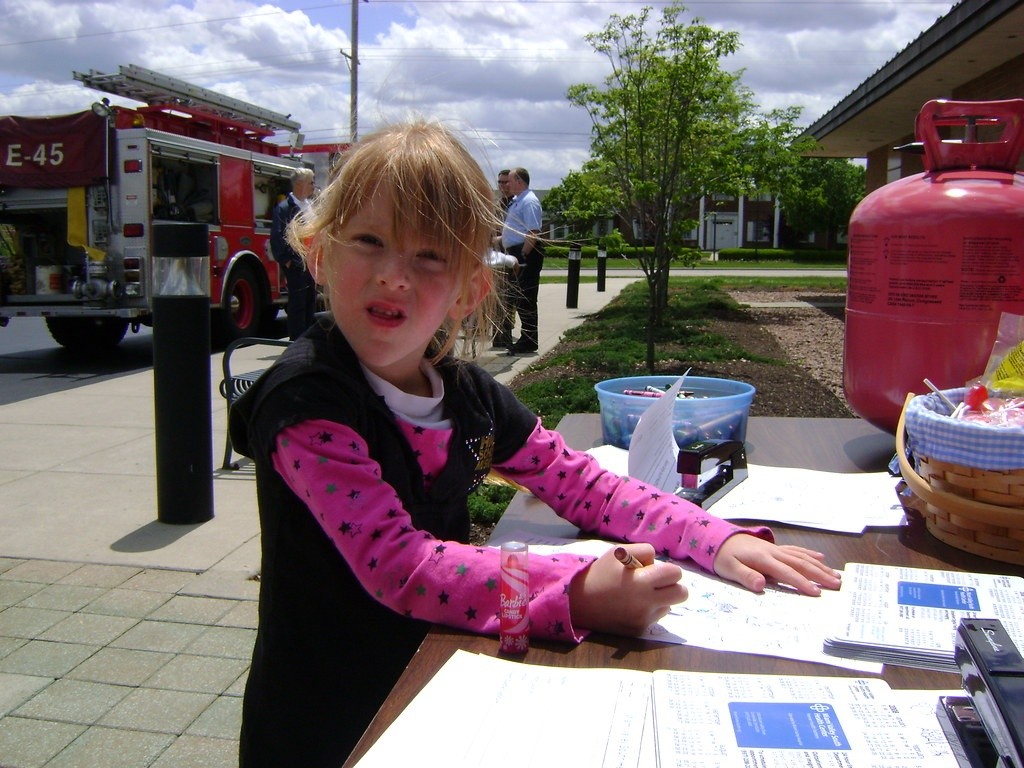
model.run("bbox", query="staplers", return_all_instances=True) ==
[677,437,750,511]
[934,617,1024,768]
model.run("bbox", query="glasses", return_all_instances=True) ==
[498,180,509,184]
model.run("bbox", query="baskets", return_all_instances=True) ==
[896,386,1024,565]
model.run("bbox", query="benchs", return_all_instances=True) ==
[220,311,334,472]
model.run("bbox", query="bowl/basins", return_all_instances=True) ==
[594,376,756,452]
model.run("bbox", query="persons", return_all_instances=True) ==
[231,121,843,767]
[492,169,543,352]
[270,168,318,340]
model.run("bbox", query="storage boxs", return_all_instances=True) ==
[888,387,1024,565]
[594,376,756,450]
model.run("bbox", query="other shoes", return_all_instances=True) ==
[507,342,537,352]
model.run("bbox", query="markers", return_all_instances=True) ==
[600,382,743,449]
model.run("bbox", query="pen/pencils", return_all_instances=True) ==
[615,546,645,571]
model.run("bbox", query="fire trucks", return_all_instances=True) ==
[0,60,358,356]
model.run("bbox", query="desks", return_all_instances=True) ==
[339,413,1024,768]
